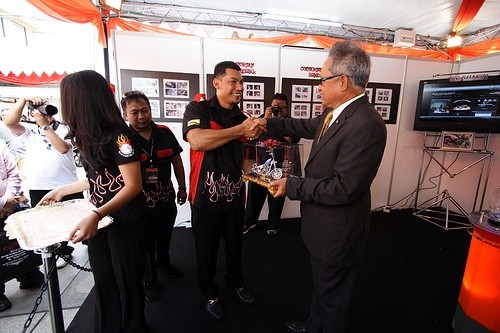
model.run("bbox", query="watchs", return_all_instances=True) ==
[42,126,49,130]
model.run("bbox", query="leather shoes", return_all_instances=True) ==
[286,319,312,333]
[233,287,256,305]
[206,298,225,319]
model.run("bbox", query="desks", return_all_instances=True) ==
[452,211,500,333]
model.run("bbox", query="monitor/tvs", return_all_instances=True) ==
[413,75,500,134]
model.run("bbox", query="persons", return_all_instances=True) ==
[181,61,267,319]
[248,43,387,333]
[0,94,84,309]
[59,70,150,333]
[244,93,301,235]
[121,94,187,302]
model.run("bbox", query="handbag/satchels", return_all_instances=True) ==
[0,217,43,284]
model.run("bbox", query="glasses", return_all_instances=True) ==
[320,73,348,85]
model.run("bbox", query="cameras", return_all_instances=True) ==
[270,105,282,116]
[28,101,58,116]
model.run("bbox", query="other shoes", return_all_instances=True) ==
[20,280,43,289]
[158,264,182,278]
[145,285,159,301]
[267,229,277,235]
[0,293,11,311]
[243,223,257,234]
[56,255,68,268]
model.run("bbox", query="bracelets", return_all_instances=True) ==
[179,188,186,191]
[92,210,102,220]
